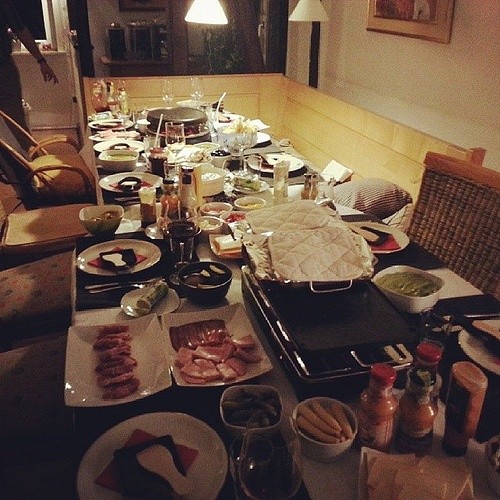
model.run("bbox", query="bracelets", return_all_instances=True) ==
[37,57,46,64]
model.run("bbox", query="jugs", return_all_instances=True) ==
[220,385,303,499]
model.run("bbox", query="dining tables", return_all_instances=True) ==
[71,107,499,500]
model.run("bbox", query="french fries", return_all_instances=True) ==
[295,401,353,445]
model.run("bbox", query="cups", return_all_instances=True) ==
[293,397,359,464]
[418,307,454,352]
[232,154,243,171]
[161,220,201,264]
[138,186,157,229]
[244,155,262,179]
[144,137,160,169]
[309,174,335,203]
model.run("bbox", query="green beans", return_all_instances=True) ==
[222,387,280,428]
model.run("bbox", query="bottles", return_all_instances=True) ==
[160,178,179,220]
[357,343,487,457]
[179,165,199,232]
[300,173,317,199]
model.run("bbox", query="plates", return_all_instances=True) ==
[64,100,410,500]
[458,320,500,376]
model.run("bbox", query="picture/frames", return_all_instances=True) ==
[364,0,455,45]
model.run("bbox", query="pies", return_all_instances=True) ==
[135,282,168,313]
[233,177,262,191]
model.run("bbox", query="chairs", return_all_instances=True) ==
[402,150,500,301]
[1,111,96,499]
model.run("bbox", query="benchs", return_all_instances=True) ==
[80,73,484,230]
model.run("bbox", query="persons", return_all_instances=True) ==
[0,0,59,152]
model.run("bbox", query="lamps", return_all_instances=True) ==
[184,0,229,25]
[287,0,329,22]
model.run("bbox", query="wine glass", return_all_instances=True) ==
[161,80,176,109]
[165,122,186,182]
[190,77,205,109]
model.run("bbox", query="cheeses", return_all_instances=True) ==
[364,452,473,500]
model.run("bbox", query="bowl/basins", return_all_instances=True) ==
[201,168,227,197]
[97,150,139,173]
[195,216,224,243]
[216,124,258,152]
[79,205,124,236]
[370,264,445,313]
[200,202,233,219]
[233,197,267,212]
[218,211,248,235]
[208,150,232,168]
[177,261,233,306]
